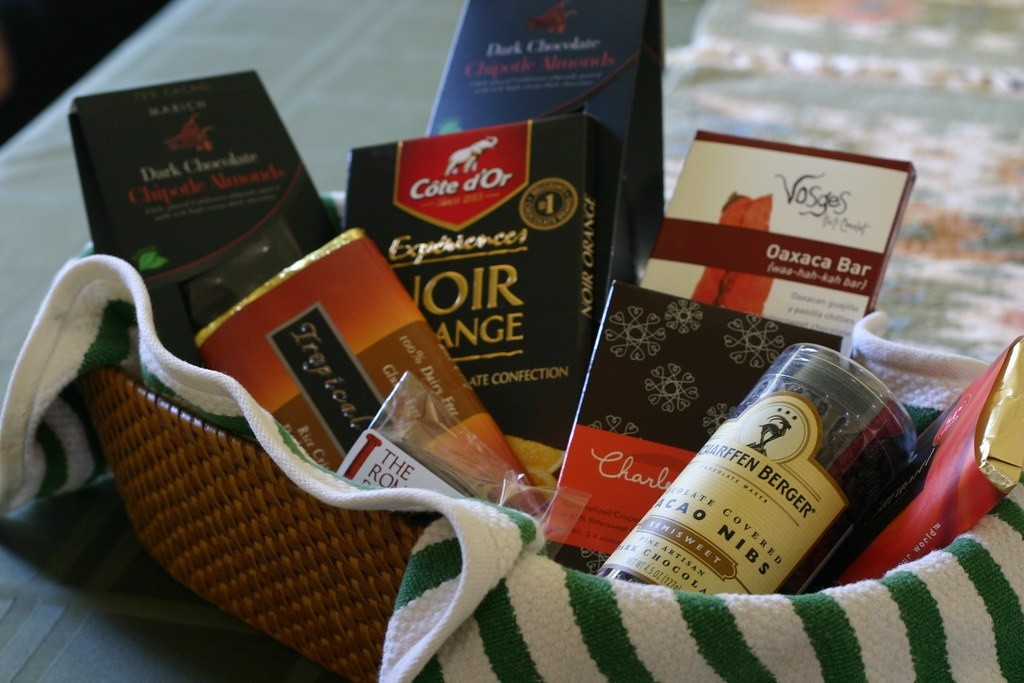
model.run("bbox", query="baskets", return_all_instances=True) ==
[82,360,427,683]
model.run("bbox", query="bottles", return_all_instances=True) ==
[595,343,918,598]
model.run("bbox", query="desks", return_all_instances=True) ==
[0,0,1024,683]
